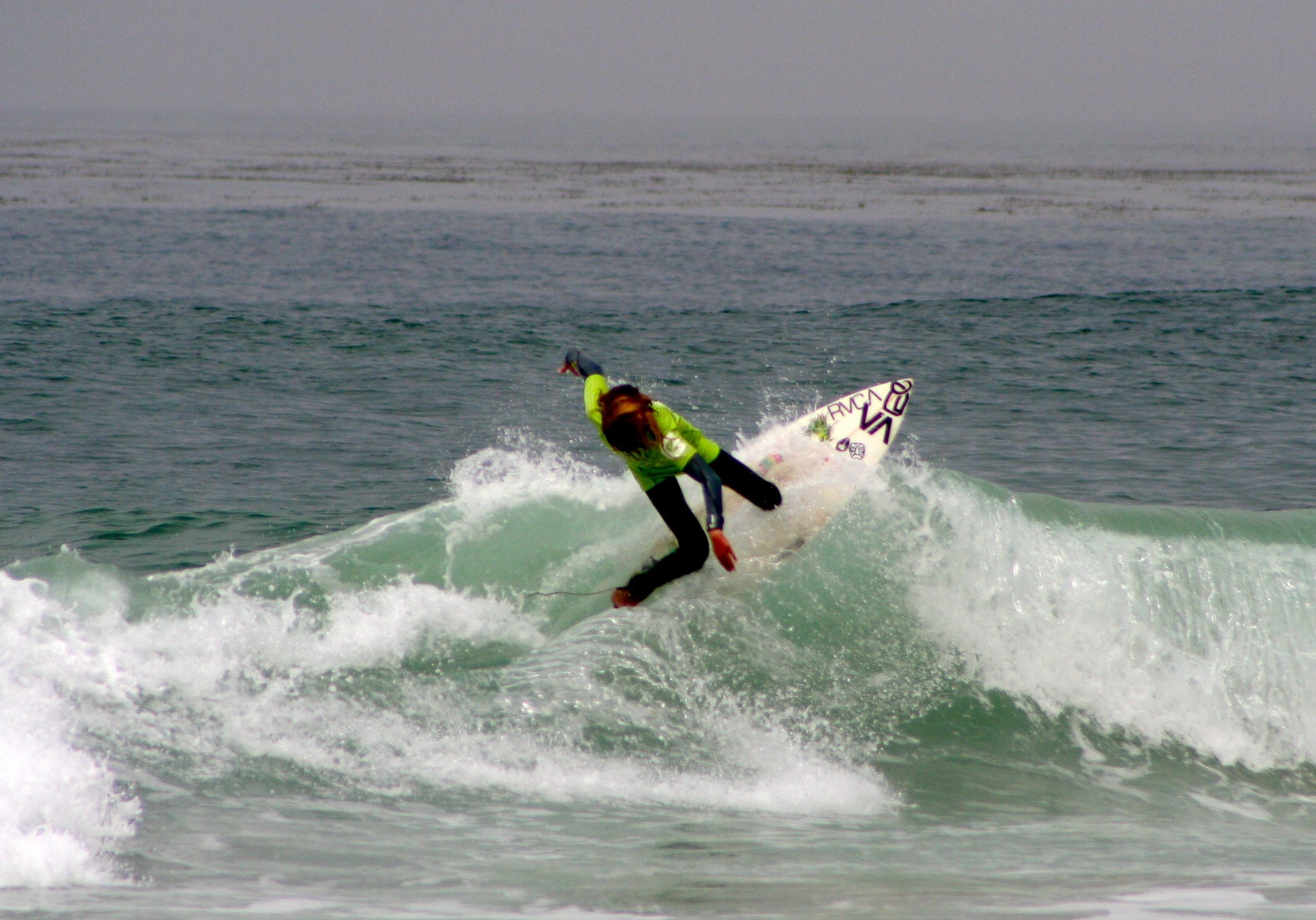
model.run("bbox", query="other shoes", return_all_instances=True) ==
[611,588,639,609]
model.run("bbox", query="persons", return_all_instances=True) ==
[560,352,738,606]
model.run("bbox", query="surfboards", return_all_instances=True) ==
[620,376,914,612]
